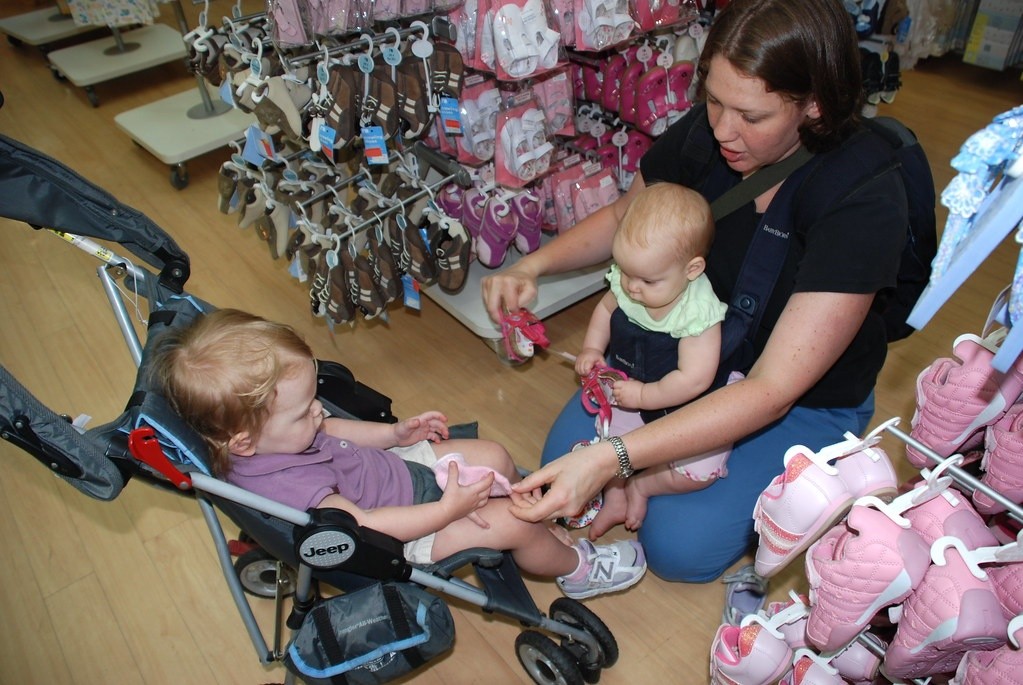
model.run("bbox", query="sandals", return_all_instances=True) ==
[496,302,551,364]
[579,362,629,425]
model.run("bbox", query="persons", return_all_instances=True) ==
[477,0,912,587]
[573,180,746,542]
[154,307,648,601]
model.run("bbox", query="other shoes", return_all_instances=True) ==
[190,0,914,329]
[562,440,603,529]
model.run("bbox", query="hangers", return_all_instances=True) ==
[741,417,1023,685]
[185,0,689,266]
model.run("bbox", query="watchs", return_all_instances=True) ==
[610,436,635,480]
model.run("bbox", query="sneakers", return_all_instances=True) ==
[704,328,1023,685]
[555,536,647,600]
